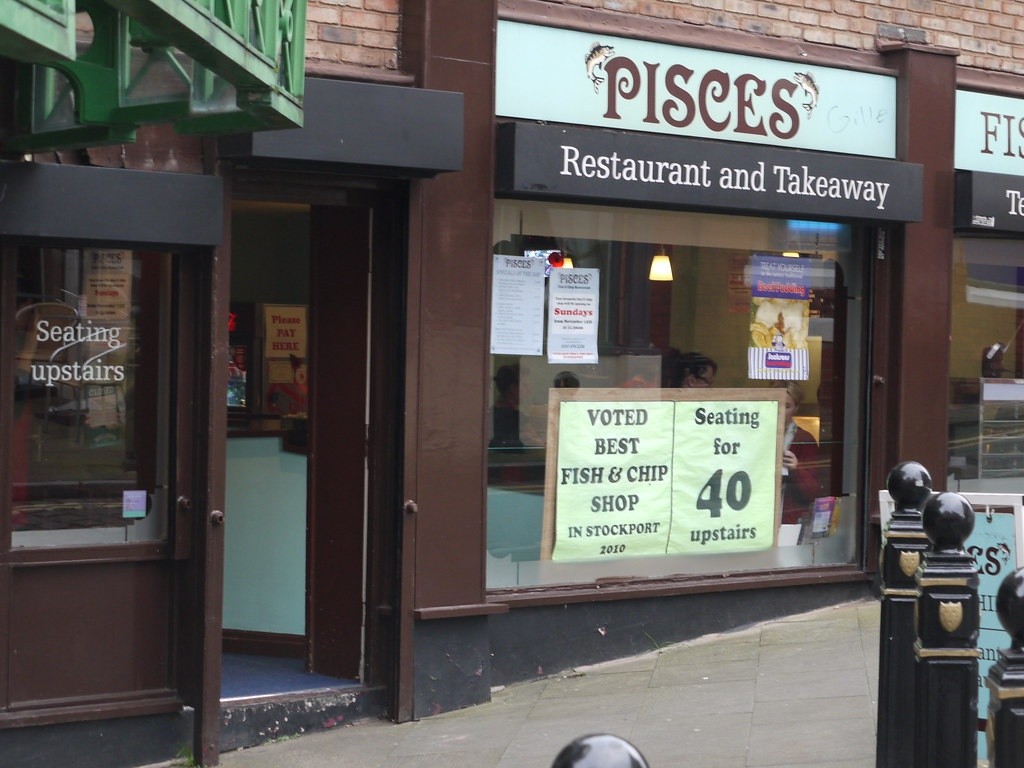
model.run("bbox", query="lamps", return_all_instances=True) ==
[561,239,573,268]
[649,245,673,281]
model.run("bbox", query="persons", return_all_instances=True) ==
[616,339,715,387]
[770,379,827,525]
[491,364,548,447]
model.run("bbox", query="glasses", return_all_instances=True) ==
[695,374,714,387]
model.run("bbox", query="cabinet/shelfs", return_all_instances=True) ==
[946,377,1024,493]
